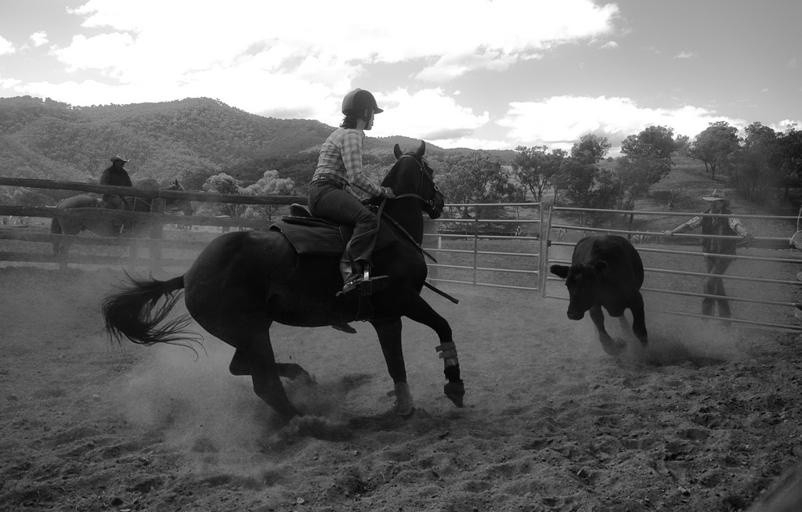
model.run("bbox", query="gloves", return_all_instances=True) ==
[381,186,396,198]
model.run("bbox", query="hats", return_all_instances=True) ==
[702,189,728,202]
[111,153,129,163]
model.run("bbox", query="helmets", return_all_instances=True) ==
[342,89,382,114]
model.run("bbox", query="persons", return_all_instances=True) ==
[309,88,395,291]
[665,189,751,327]
[100,156,132,228]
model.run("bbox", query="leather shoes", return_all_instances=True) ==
[341,261,361,283]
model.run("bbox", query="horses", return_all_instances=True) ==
[102,141,464,421]
[51,178,194,270]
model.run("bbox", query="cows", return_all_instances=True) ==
[550,235,648,355]
